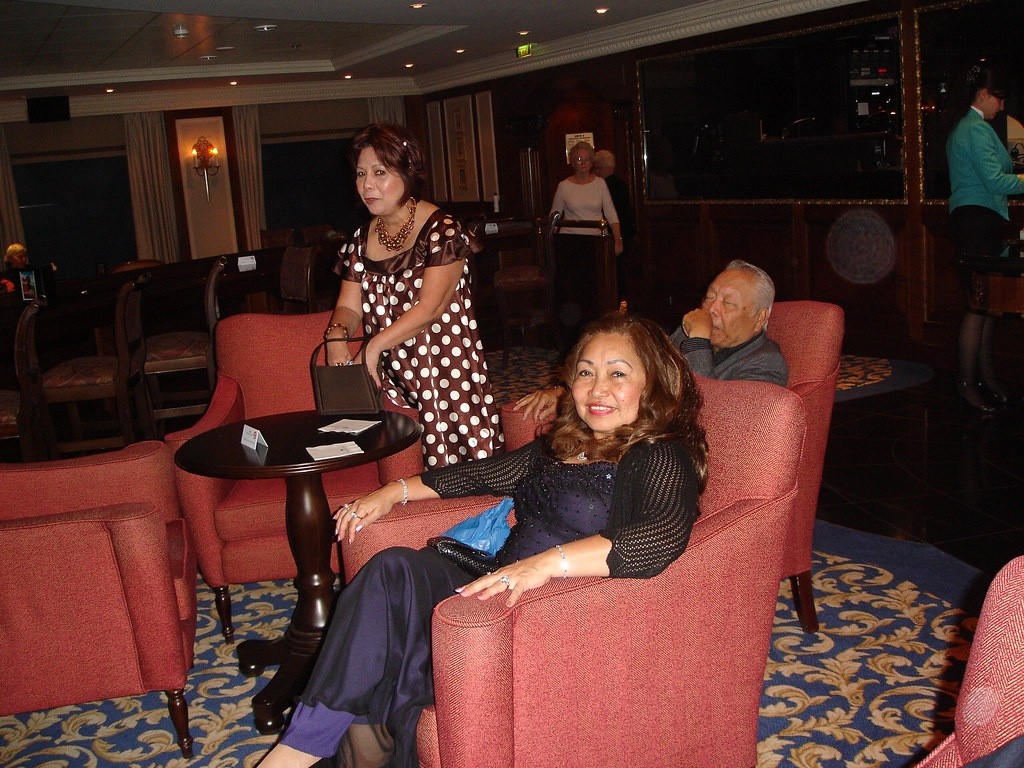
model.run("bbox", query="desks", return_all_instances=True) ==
[175,410,420,735]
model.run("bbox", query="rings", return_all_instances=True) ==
[350,512,363,520]
[335,363,343,367]
[500,575,509,586]
[485,571,491,578]
[342,503,353,512]
[348,360,354,365]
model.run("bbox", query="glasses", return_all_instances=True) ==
[577,157,593,161]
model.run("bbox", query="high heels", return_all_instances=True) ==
[952,375,1001,418]
[976,375,1008,402]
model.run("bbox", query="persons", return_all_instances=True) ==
[251,313,712,768]
[322,120,507,475]
[548,142,637,314]
[943,58,1024,419]
[23,276,35,300]
[512,258,790,424]
[0,243,57,294]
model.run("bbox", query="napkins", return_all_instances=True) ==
[305,441,364,460]
[318,419,381,433]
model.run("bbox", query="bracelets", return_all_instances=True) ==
[616,236,623,240]
[397,478,409,506]
[324,323,350,342]
[556,545,568,581]
[553,384,565,393]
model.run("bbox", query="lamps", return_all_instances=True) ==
[194,137,221,203]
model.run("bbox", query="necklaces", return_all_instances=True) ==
[374,196,417,252]
[577,448,589,462]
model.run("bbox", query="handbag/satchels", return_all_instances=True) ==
[310,337,385,415]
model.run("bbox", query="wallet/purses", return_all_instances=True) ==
[426,536,504,578]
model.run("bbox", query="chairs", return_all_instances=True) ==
[0,217,1024,768]
